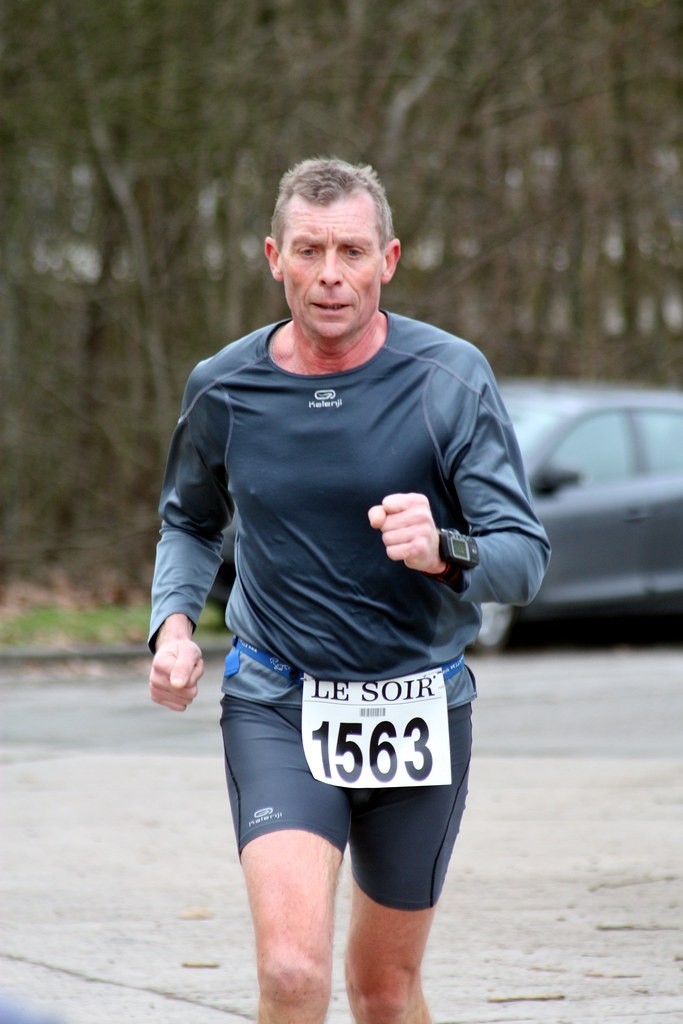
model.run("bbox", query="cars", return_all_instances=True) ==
[198,381,683,654]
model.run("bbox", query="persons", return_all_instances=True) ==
[148,161,555,1024]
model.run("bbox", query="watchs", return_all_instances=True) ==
[429,530,480,583]
